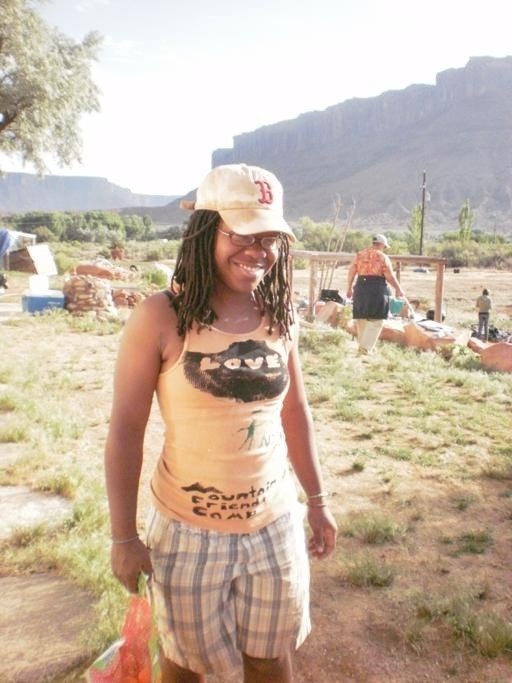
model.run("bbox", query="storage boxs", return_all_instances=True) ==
[22,290,66,315]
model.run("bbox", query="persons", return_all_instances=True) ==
[106,164,338,683]
[474,287,493,342]
[345,234,405,356]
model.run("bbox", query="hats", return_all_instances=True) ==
[373,234,389,249]
[181,163,297,243]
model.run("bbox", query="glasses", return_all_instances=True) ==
[216,228,283,250]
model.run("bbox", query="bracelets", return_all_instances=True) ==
[108,534,140,546]
[304,499,329,507]
[306,487,331,500]
